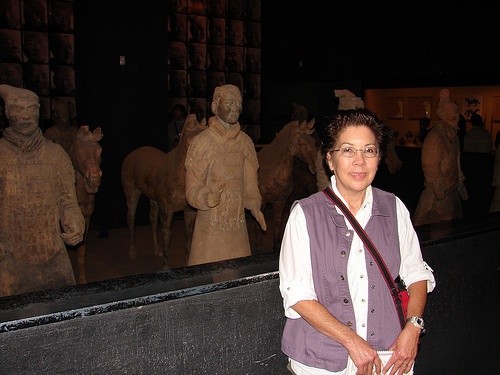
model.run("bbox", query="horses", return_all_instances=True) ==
[382,137,402,174]
[121,113,209,271]
[244,118,317,253]
[42,125,103,285]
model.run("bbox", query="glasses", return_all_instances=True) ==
[327,145,382,159]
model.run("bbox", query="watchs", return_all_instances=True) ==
[406,316,425,330]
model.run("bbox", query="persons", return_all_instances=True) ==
[0,84,86,299]
[410,88,469,229]
[184,83,268,270]
[168,102,186,150]
[288,89,365,231]
[416,111,500,213]
[278,109,436,375]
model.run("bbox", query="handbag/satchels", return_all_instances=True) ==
[392,279,427,353]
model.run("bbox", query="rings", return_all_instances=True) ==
[403,363,409,366]
